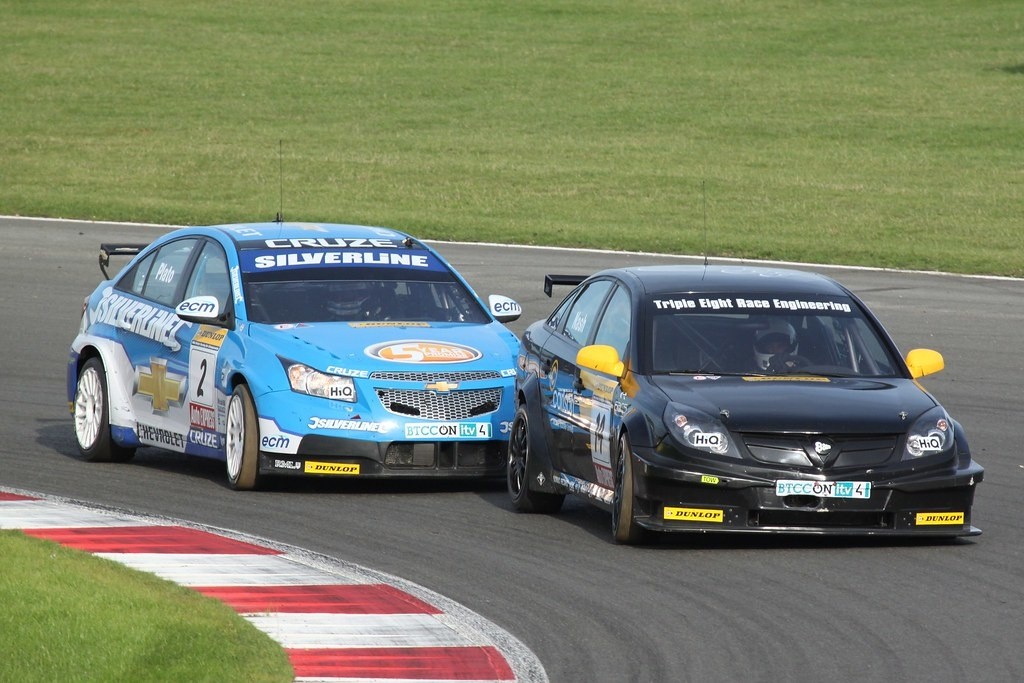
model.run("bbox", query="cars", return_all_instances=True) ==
[505,263,988,546]
[66,221,523,492]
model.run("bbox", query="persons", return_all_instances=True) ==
[325,270,385,322]
[749,318,799,374]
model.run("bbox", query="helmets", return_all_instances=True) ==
[326,281,382,320]
[753,320,799,373]
